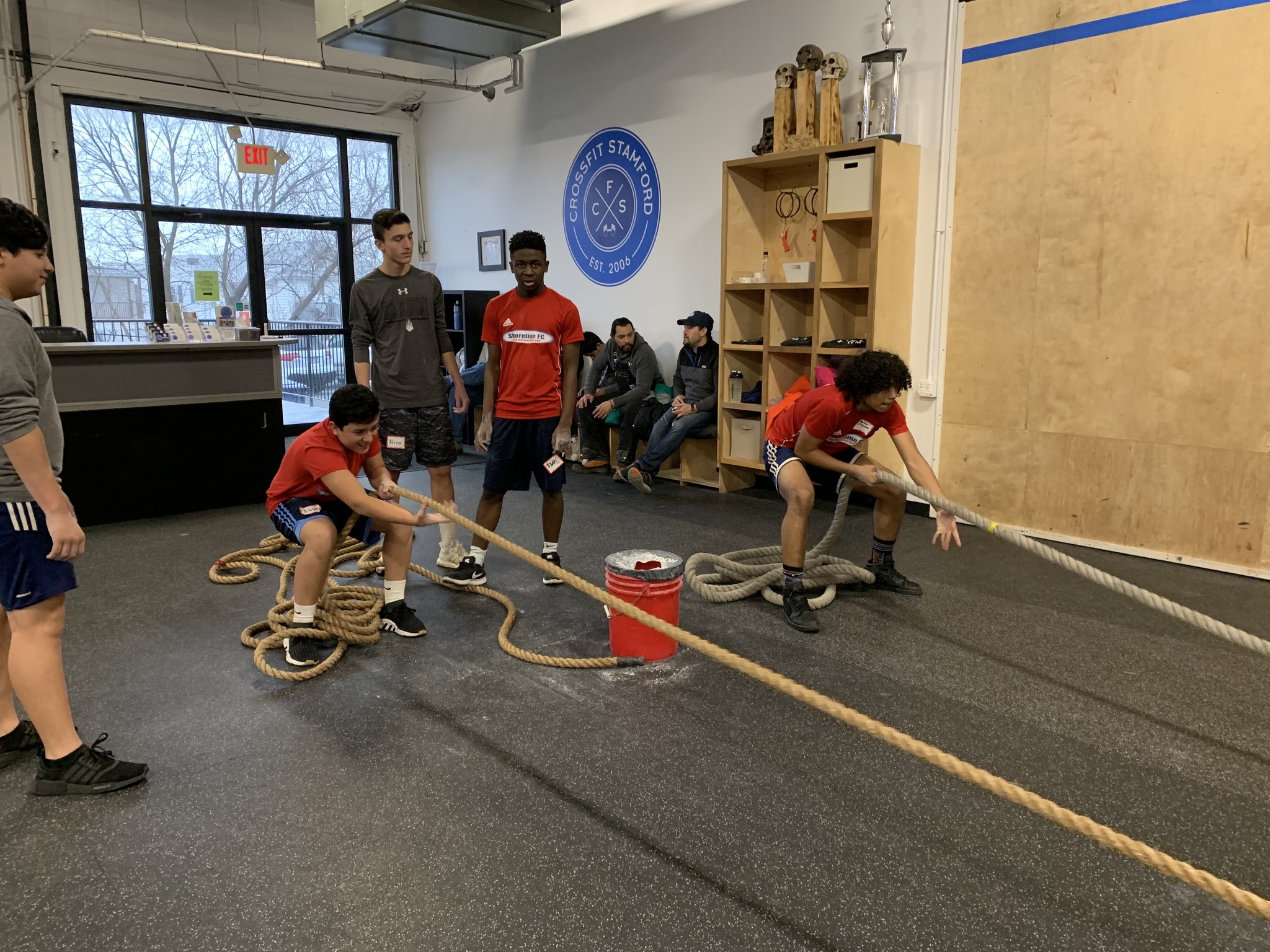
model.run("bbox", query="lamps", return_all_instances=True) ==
[227,125,289,174]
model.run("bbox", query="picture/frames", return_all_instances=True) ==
[476,229,507,272]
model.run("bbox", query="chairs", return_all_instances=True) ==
[32,325,89,343]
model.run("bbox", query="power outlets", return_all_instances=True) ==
[917,378,937,397]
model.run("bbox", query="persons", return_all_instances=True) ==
[619,310,719,494]
[569,331,606,465]
[443,342,489,457]
[441,230,585,586]
[349,208,470,577]
[266,383,456,666]
[0,197,148,794]
[763,349,961,632]
[571,317,664,481]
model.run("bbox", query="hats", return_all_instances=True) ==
[677,310,714,329]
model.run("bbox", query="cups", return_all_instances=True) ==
[729,370,743,403]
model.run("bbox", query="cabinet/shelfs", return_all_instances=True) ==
[568,135,923,498]
[442,289,500,444]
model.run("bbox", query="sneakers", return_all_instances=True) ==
[618,459,641,482]
[540,551,566,584]
[782,581,821,632]
[861,559,923,595]
[282,622,319,666]
[376,598,426,637]
[30,731,150,797]
[0,719,79,769]
[627,467,653,494]
[570,436,579,461]
[436,540,469,568]
[571,455,609,475]
[442,556,487,585]
[613,466,625,481]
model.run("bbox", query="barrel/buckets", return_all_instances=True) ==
[603,566,683,662]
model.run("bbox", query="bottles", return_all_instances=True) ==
[454,301,462,330]
[761,252,771,283]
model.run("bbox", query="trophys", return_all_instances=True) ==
[856,0,907,142]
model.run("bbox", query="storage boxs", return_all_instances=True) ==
[780,261,816,283]
[826,152,875,214]
[729,417,761,462]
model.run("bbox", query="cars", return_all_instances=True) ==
[322,334,374,385]
[274,335,338,391]
